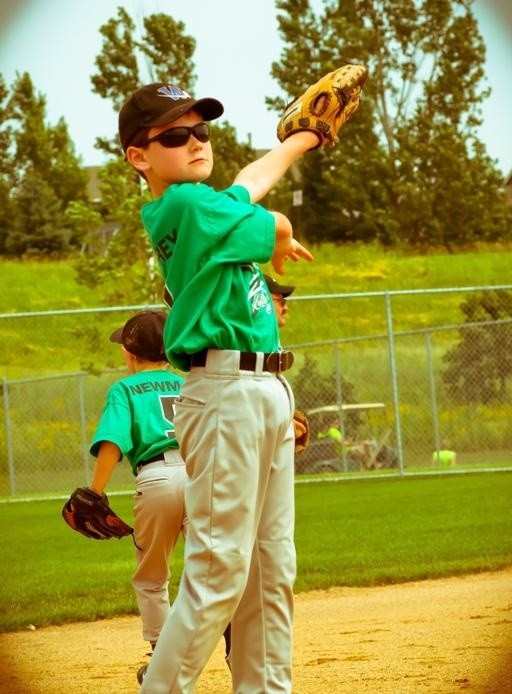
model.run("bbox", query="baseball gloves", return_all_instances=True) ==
[62,487,134,540]
[277,65,366,148]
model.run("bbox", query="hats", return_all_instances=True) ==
[109,310,168,364]
[262,273,295,298]
[116,80,224,160]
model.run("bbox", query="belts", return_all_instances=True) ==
[136,449,165,469]
[189,348,295,374]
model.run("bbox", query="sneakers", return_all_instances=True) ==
[135,641,158,686]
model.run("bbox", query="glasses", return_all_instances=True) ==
[124,122,212,161]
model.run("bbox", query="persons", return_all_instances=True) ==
[260,276,294,332]
[430,436,457,465]
[319,415,384,470]
[59,309,237,687]
[117,54,372,694]
